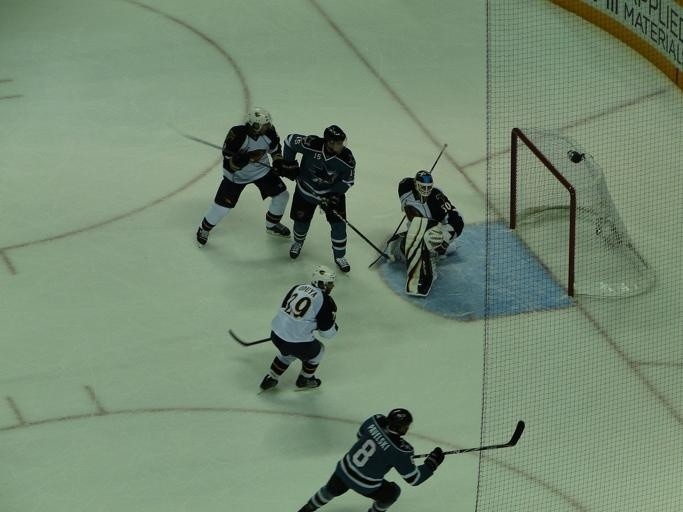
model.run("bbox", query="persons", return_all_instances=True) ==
[387,170,465,298]
[280,124,356,273]
[298,408,445,512]
[259,266,340,389]
[196,106,291,245]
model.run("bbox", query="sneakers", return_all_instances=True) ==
[334,254,350,272]
[260,374,278,390]
[297,374,321,388]
[266,221,291,236]
[197,226,209,245]
[290,240,303,258]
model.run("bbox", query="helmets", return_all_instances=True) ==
[415,171,434,196]
[247,108,271,132]
[325,125,346,145]
[311,265,337,293]
[388,409,413,435]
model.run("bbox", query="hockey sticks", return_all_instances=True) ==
[366,142,449,269]
[230,331,271,346]
[413,420,525,459]
[166,123,279,170]
[323,203,404,262]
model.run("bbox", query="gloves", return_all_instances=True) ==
[424,447,445,471]
[322,193,341,212]
[273,158,287,177]
[229,151,251,171]
[284,159,300,181]
